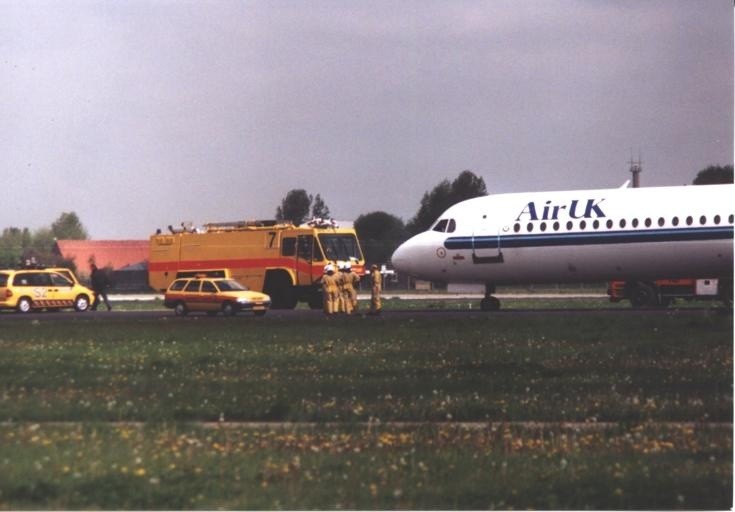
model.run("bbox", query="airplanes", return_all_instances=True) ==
[390,178,734,310]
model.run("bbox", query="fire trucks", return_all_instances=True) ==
[604,279,729,307]
[147,218,366,309]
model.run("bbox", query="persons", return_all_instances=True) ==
[321,260,382,314]
[89,264,112,311]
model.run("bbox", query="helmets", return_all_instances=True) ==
[323,261,351,272]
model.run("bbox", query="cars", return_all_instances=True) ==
[164,273,272,316]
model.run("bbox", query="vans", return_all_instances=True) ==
[52,267,78,282]
[1,269,97,314]
